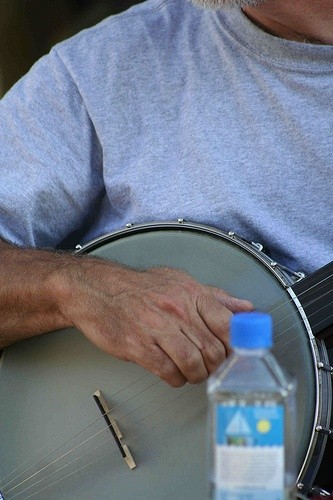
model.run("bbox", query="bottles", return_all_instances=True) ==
[206,310,296,500]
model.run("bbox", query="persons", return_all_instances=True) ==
[2,0,333,386]
